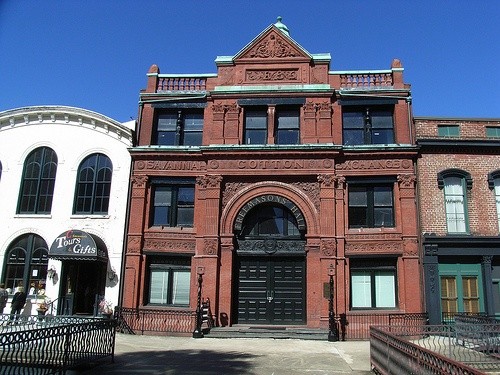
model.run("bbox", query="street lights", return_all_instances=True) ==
[326,262,338,342]
[193,266,207,339]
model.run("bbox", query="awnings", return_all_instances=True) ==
[46,229,109,264]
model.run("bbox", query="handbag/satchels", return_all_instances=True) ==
[2,289,7,308]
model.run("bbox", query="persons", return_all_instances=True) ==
[0,282,8,314]
[7,286,26,326]
